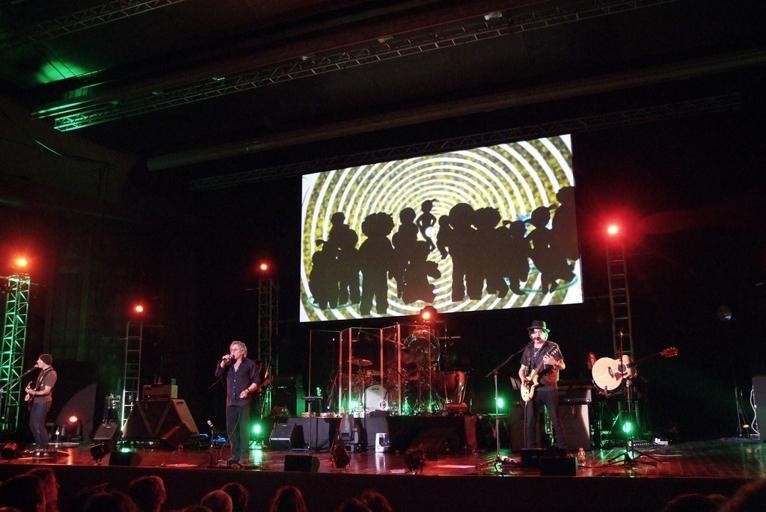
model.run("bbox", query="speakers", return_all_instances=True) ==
[109,450,141,466]
[284,454,320,472]
[538,456,579,475]
[268,423,298,448]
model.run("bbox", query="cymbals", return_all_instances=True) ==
[348,357,372,367]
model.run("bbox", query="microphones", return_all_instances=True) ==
[619,332,623,346]
[222,355,235,361]
[24,364,39,374]
[530,335,537,343]
[207,420,215,430]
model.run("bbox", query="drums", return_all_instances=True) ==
[362,384,389,412]
[404,332,441,368]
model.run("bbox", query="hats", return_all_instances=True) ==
[528,320,550,332]
[40,354,52,365]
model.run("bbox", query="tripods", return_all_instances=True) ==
[475,374,507,470]
[606,348,662,465]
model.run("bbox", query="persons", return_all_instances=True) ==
[215,341,260,465]
[272,486,308,512]
[183,482,248,512]
[308,187,575,315]
[336,488,390,512]
[519,321,568,450]
[76,475,166,512]
[25,354,58,450]
[2,467,64,512]
[662,478,765,512]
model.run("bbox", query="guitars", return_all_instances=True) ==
[592,347,678,395]
[521,345,559,402]
